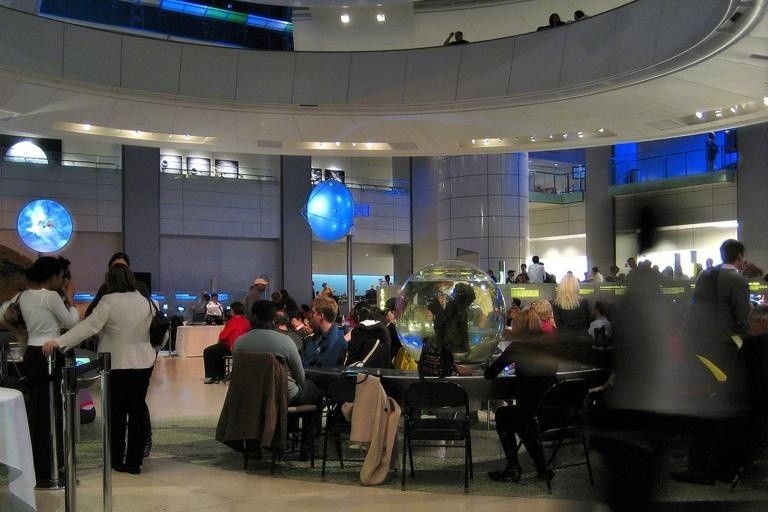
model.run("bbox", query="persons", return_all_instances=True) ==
[42,263,169,475]
[311,169,321,184]
[705,134,719,171]
[329,170,344,183]
[52,256,77,368]
[189,239,767,511]
[0,257,79,488]
[444,31,470,46]
[565,9,588,25]
[537,13,566,32]
[85,252,152,459]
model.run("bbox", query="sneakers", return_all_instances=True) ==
[111,460,140,475]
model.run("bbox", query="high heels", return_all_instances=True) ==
[489,466,555,482]
[204,377,220,384]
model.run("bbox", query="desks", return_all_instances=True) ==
[0,386,38,512]
[175,324,225,358]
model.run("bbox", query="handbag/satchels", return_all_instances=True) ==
[4,289,24,328]
[418,337,454,376]
[147,296,169,346]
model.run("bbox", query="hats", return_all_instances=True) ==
[255,278,268,286]
